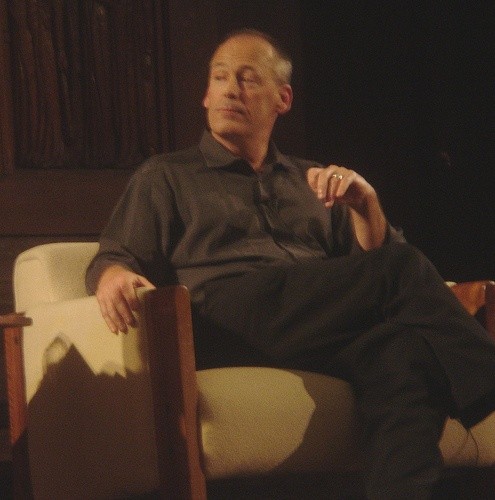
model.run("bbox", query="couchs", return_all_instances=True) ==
[0,241,494,500]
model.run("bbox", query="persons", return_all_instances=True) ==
[83,27,493,499]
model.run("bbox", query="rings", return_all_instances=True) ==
[332,173,343,179]
